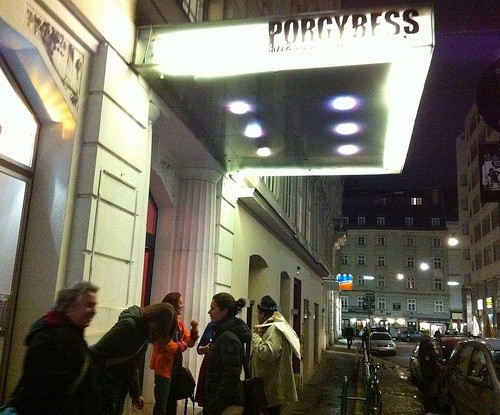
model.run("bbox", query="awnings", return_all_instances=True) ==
[417,313,449,320]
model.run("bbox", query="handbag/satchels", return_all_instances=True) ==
[235,377,269,408]
[171,342,196,400]
[254,312,302,361]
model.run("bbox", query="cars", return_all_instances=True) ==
[368,332,397,355]
[390,327,424,342]
[409,332,500,415]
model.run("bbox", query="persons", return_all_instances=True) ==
[420,335,437,397]
[361,329,369,348]
[70,302,178,415]
[194,292,252,415]
[344,325,354,350]
[242,295,299,415]
[150,292,199,415]
[0,281,100,415]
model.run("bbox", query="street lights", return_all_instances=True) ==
[363,274,375,330]
[448,221,487,339]
[398,273,418,333]
[419,256,459,334]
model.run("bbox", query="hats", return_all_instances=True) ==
[257,295,278,312]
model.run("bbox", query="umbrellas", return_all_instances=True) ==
[449,318,453,333]
[472,316,479,339]
[457,318,461,333]
[468,319,472,338]
